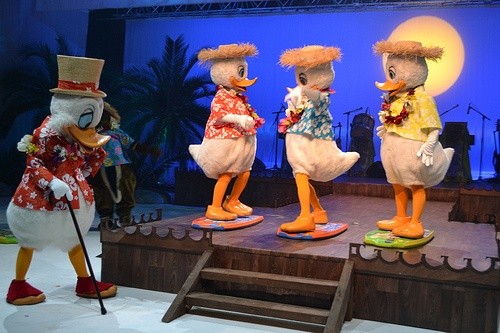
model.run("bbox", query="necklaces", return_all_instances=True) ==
[278,98,308,133]
[379,89,416,124]
[237,93,265,129]
[17,134,82,162]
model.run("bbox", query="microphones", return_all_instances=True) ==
[467,105,471,114]
[366,107,368,114]
[281,102,286,110]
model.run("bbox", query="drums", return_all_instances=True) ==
[350,113,374,137]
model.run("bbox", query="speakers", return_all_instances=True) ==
[437,135,473,184]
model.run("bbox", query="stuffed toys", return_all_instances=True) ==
[88,103,139,231]
[373,40,454,239]
[188,42,265,221]
[278,45,361,233]
[6,55,117,305]
